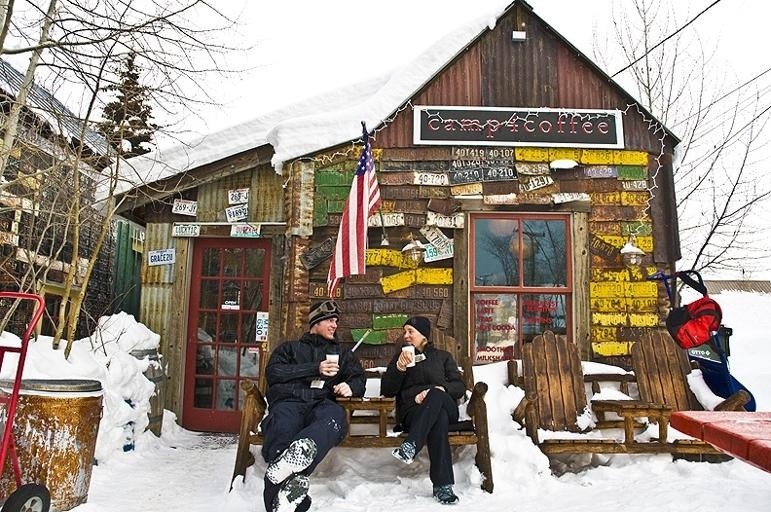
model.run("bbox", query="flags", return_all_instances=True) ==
[325,129,384,298]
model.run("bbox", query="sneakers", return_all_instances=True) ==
[392,440,416,464]
[270,477,312,511]
[264,435,318,484]
[434,482,460,505]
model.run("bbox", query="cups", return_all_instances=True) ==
[326,354,338,374]
[402,345,416,367]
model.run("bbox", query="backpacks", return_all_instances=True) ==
[664,270,724,352]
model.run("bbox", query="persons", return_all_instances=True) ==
[378,316,467,504]
[259,299,368,512]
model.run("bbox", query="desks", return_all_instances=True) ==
[671,409,771,473]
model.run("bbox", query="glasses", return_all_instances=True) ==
[310,302,339,322]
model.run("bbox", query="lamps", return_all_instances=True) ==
[620,233,646,269]
[401,231,427,269]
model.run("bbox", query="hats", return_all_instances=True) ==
[403,315,431,340]
[309,300,341,328]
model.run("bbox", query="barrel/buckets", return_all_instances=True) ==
[124,347,168,450]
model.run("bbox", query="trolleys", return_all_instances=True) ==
[646,270,757,412]
[0,292,51,511]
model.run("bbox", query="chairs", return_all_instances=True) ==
[507,329,752,455]
[229,352,493,493]
